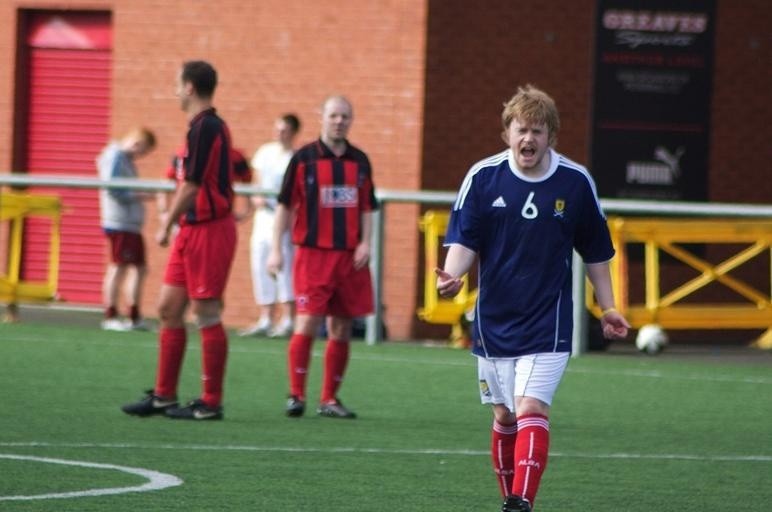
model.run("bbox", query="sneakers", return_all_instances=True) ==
[101,320,155,334]
[123,393,177,417]
[503,495,530,512]
[242,318,291,337]
[166,399,223,420]
[318,400,355,418]
[288,397,305,416]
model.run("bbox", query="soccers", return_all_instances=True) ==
[637,324,669,353]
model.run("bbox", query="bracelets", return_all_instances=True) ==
[601,307,615,318]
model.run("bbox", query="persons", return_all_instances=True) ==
[119,62,237,421]
[95,126,155,330]
[157,146,255,326]
[432,87,632,512]
[237,112,299,339]
[265,95,381,419]
